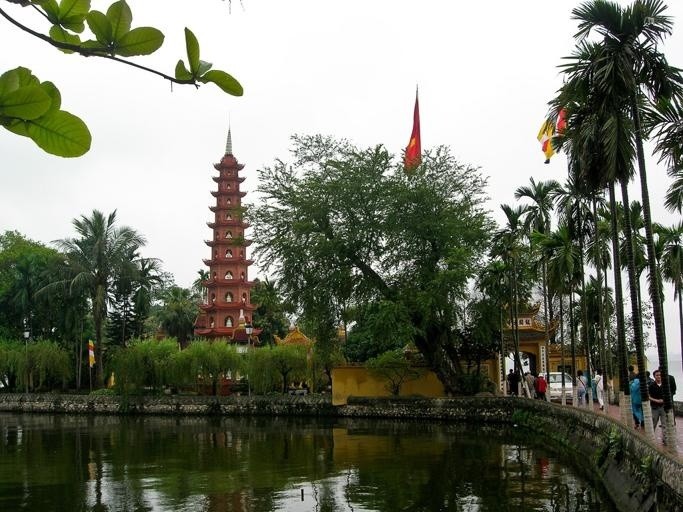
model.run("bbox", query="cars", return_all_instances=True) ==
[537,371,573,401]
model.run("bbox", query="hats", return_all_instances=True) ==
[538,373,544,378]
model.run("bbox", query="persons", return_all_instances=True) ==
[507,368,548,402]
[627,364,677,432]
[504,442,551,476]
[576,369,588,408]
[649,369,666,446]
[595,368,614,410]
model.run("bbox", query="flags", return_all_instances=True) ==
[536,78,572,165]
[88,337,96,368]
[403,89,423,181]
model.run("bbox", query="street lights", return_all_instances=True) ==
[22,310,33,343]
[245,322,253,398]
